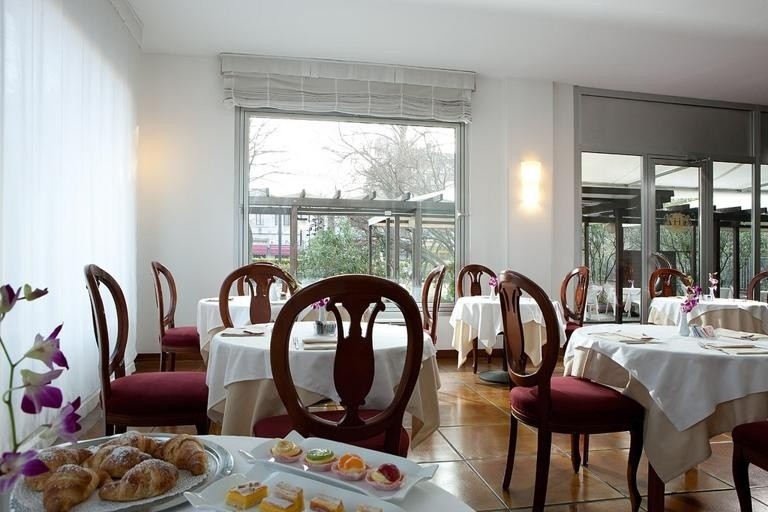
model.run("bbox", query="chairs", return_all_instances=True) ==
[731,423,767,507]
[86,262,213,437]
[747,271,767,300]
[149,261,208,371]
[649,269,692,299]
[452,265,505,372]
[583,285,641,323]
[252,271,427,460]
[217,260,296,330]
[542,266,589,361]
[496,268,640,511]
[423,262,448,342]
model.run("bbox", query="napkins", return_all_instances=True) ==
[588,331,652,344]
[713,328,766,340]
[705,341,766,356]
[302,336,338,349]
[220,325,263,337]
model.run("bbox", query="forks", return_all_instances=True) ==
[292,337,301,351]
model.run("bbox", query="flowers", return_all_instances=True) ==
[0,283,82,494]
[310,298,328,309]
[627,280,634,283]
[681,285,702,313]
[708,273,719,292]
[489,277,497,286]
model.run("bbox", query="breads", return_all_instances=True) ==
[24,430,208,512]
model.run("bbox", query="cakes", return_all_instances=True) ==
[227,440,404,512]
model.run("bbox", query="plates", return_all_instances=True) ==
[182,430,439,512]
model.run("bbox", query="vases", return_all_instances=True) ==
[676,312,688,337]
[318,306,326,322]
[269,284,278,300]
[489,286,495,301]
[709,287,715,299]
[631,283,634,288]
